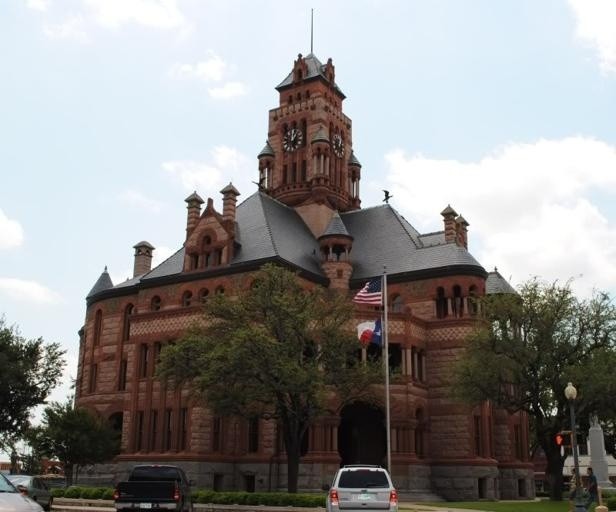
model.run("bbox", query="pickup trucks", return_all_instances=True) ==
[110,464,195,512]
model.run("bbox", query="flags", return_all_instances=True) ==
[356,316,381,347]
[345,276,384,305]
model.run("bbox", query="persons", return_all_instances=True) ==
[568,467,582,501]
[585,468,600,510]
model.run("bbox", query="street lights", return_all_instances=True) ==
[561,380,587,512]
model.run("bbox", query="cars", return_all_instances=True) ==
[0,472,43,512]
[6,473,53,509]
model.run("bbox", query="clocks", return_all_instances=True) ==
[331,135,345,159]
[281,129,304,153]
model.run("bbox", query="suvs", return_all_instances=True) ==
[321,465,399,512]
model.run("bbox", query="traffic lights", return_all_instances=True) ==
[556,430,571,447]
[574,432,585,446]
